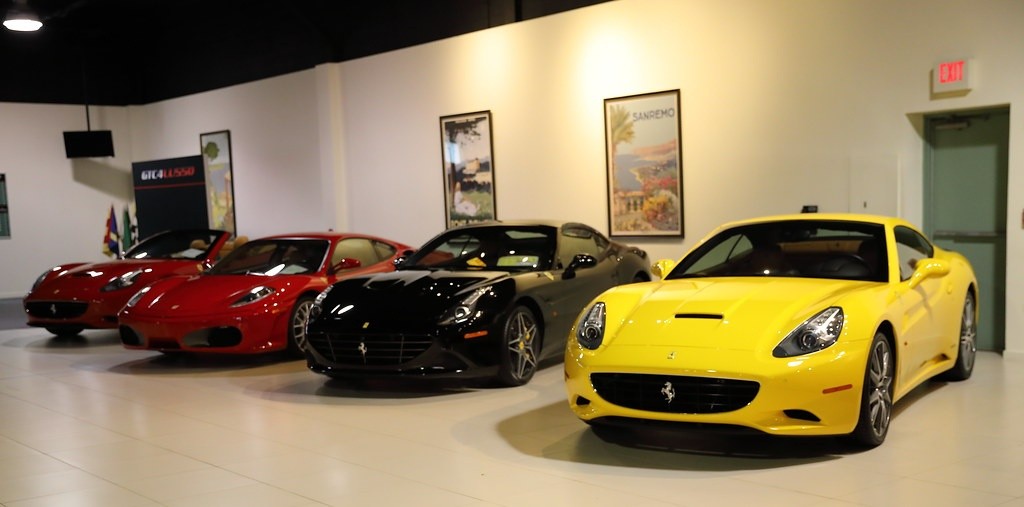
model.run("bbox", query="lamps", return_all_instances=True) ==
[1,0,43,32]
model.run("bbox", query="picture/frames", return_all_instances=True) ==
[604,89,685,239]
[199,130,237,239]
[440,110,497,230]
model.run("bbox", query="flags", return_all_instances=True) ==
[121,208,138,252]
[101,206,119,256]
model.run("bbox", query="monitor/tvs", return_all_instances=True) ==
[63,131,113,158]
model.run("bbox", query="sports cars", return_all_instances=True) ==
[20,228,252,337]
[564,211,980,451]
[305,219,651,386]
[116,227,458,361]
